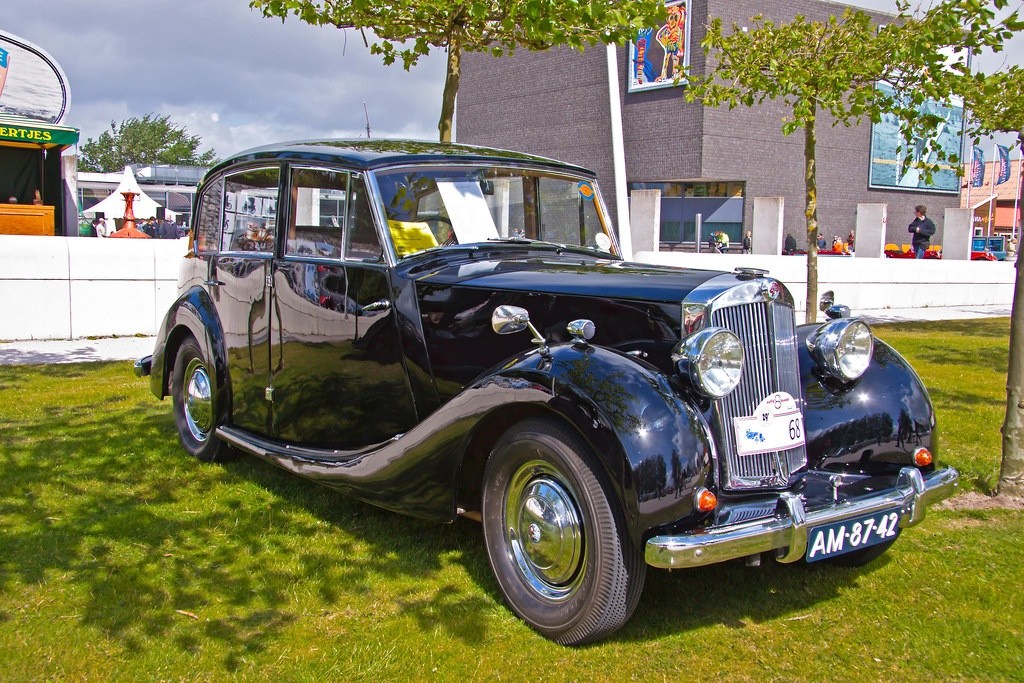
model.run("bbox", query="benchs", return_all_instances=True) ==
[235,223,379,257]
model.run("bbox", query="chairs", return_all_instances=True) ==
[885,242,942,258]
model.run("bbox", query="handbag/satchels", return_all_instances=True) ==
[921,241,930,250]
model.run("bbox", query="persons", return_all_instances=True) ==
[743,231,752,254]
[908,205,936,259]
[832,235,843,254]
[817,233,826,250]
[513,228,525,238]
[847,230,855,248]
[785,233,796,256]
[136,214,180,240]
[709,230,729,254]
[91,218,106,238]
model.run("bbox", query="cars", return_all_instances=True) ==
[133,136,961,649]
